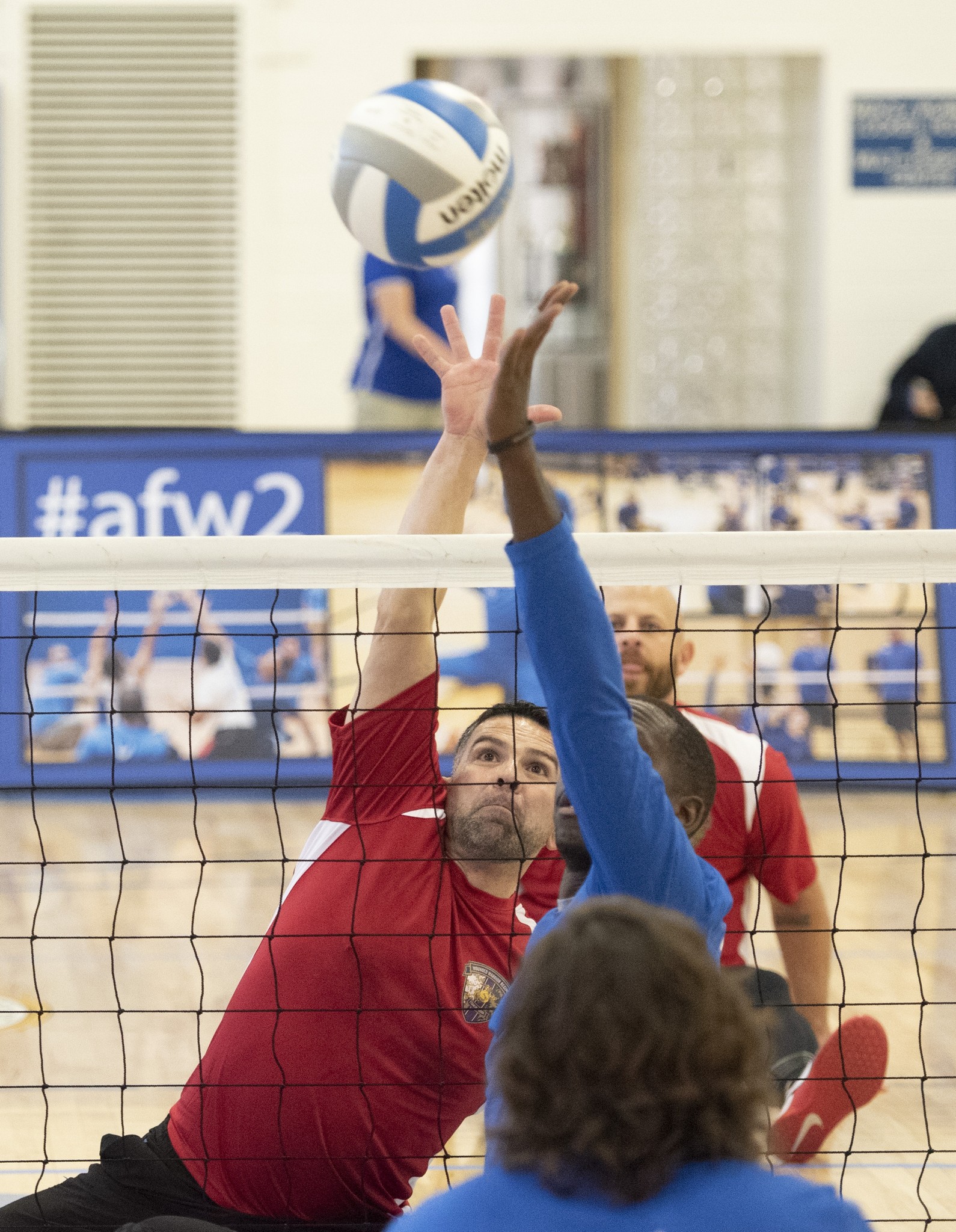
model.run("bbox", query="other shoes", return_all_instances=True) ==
[766,1015,888,1163]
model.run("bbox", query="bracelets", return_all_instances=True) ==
[486,418,535,456]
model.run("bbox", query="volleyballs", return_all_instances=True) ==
[324,78,519,270]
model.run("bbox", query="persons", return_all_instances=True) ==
[487,278,731,1167]
[39,580,330,773]
[597,580,834,1048]
[611,474,929,763]
[390,893,871,1232]
[0,291,569,1232]
[881,320,956,435]
